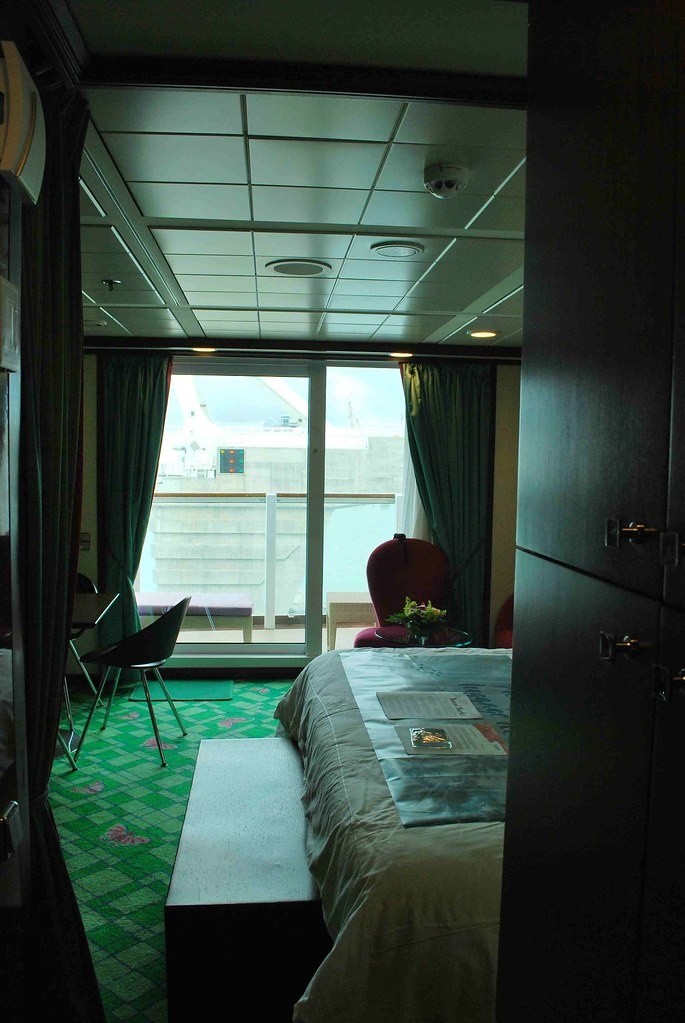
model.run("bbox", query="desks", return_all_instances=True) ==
[63,594,122,731]
[373,625,473,647]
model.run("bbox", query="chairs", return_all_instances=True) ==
[73,594,192,767]
[353,538,452,647]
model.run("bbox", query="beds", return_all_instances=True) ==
[273,647,515,1023]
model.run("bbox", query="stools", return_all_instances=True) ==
[326,592,377,651]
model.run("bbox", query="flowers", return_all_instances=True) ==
[403,597,446,625]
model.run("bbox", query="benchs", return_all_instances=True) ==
[134,592,253,643]
[165,736,324,1023]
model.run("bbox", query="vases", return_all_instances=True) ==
[414,621,433,629]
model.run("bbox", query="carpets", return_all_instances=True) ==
[126,679,233,701]
